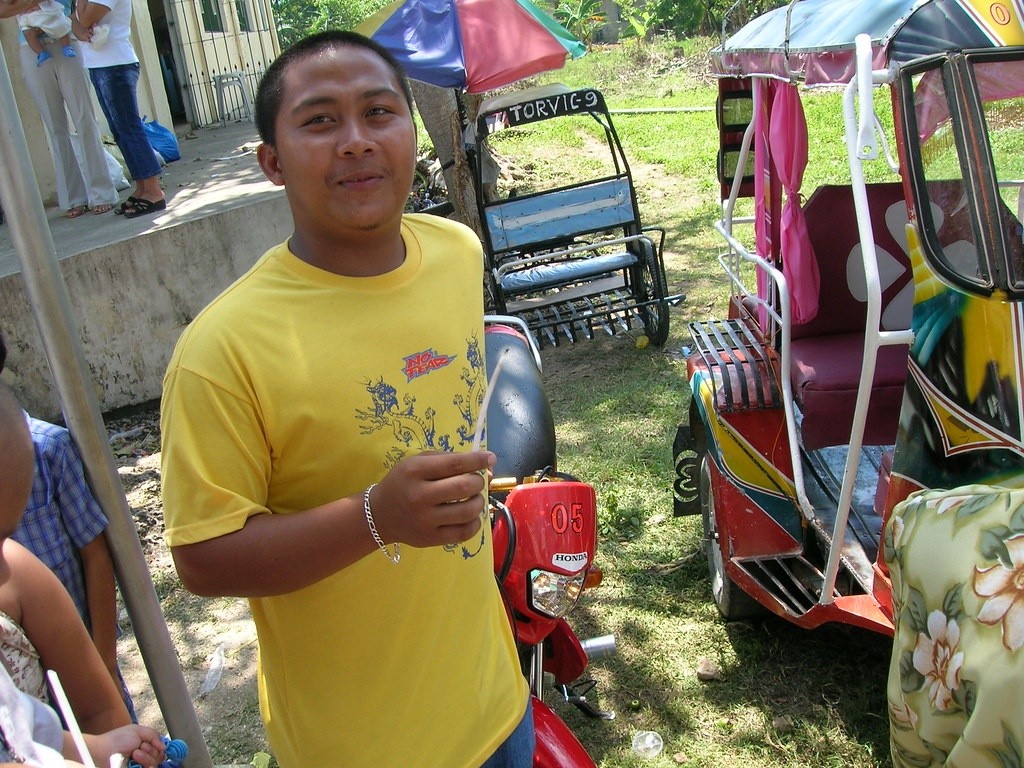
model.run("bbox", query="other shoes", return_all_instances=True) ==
[37,50,53,67]
[63,45,77,59]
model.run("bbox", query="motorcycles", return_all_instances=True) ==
[665,1,1024,646]
[482,313,618,768]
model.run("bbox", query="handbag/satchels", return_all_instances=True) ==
[93,115,181,191]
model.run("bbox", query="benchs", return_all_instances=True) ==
[774,180,1024,452]
[484,177,644,299]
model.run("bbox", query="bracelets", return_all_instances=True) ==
[365,483,401,564]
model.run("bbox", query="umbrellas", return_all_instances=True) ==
[352,0,590,94]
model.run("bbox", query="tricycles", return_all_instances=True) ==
[350,0,686,356]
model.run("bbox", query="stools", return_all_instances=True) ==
[212,71,255,126]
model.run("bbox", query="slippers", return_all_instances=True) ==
[114,196,137,215]
[124,198,166,218]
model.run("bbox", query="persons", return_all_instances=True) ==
[158,30,536,768]
[0,0,167,217]
[0,335,181,768]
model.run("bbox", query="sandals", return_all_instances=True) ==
[67,205,89,218]
[92,204,113,215]
